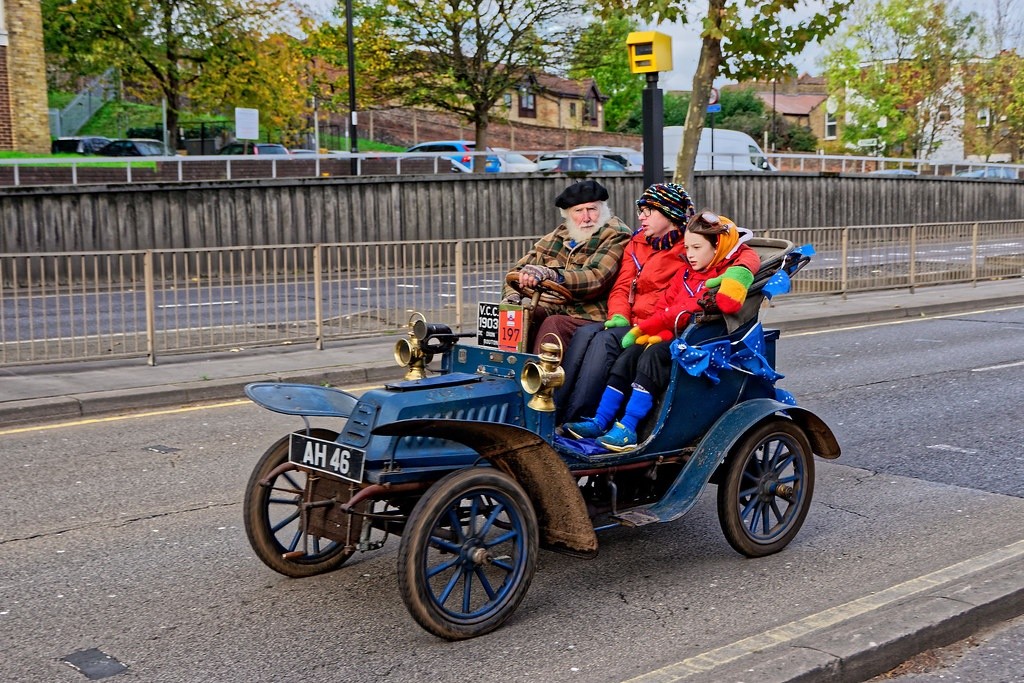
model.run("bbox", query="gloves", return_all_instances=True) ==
[604,314,630,327]
[621,325,644,348]
[643,335,662,352]
[705,266,755,314]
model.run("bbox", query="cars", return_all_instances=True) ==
[53,136,111,156]
[242,238,841,641]
[215,142,289,155]
[406,141,502,172]
[93,138,183,156]
[489,146,537,172]
[531,153,627,171]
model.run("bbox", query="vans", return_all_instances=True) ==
[663,127,778,171]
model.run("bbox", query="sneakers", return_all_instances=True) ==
[563,416,608,440]
[595,421,637,453]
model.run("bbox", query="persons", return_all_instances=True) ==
[552,183,759,436]
[500,180,633,362]
[561,210,738,453]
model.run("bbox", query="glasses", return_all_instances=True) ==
[636,206,653,217]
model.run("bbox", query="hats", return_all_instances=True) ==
[555,180,609,210]
[636,183,695,228]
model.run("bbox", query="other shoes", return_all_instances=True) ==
[555,424,569,438]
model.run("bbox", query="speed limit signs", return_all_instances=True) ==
[709,88,718,104]
